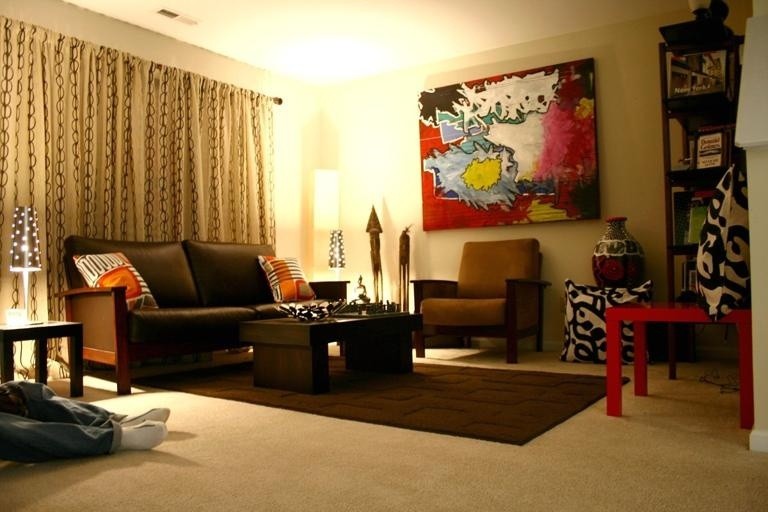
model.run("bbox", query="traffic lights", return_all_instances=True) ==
[71,252,159,310]
[560,278,656,369]
[257,252,318,302]
[691,164,746,319]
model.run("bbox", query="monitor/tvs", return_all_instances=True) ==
[686,121,734,169]
[676,187,719,245]
[663,47,727,95]
[677,254,701,298]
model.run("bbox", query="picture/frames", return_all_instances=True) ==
[310,281,350,304]
[603,298,752,428]
[0,318,86,399]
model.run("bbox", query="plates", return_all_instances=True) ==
[655,32,744,308]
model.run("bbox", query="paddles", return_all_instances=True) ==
[129,359,629,446]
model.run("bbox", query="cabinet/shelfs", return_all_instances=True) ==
[591,215,647,286]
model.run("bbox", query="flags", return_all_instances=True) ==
[589,214,645,290]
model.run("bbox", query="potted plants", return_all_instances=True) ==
[692,128,728,172]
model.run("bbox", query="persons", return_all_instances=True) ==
[0,378,171,460]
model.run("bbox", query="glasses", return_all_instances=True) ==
[9,205,43,326]
[328,230,345,281]
[311,166,342,281]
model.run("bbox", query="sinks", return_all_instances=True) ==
[57,231,350,395]
[406,237,551,366]
